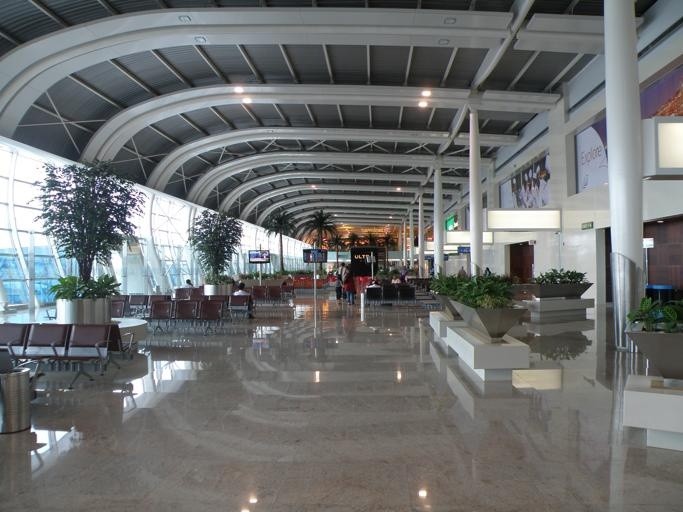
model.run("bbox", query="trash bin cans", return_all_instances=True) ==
[0,367,31,434]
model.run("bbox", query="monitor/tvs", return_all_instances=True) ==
[303,249,327,263]
[249,250,270,263]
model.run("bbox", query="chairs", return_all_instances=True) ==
[366,283,416,306]
[0,283,295,399]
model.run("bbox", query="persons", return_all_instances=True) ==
[334,274,342,300]
[337,262,348,282]
[232,282,256,319]
[366,265,402,307]
[342,273,356,305]
[483,266,491,277]
[342,306,355,344]
[182,279,193,288]
[510,165,548,208]
[335,306,341,338]
[457,266,466,278]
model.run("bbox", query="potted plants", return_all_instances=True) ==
[624,296,683,389]
[429,268,594,344]
